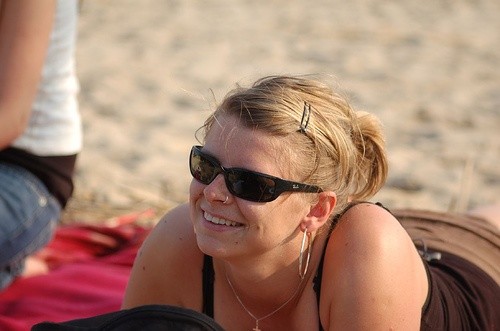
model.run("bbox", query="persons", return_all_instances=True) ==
[1,0,81,296]
[120,77,500,331]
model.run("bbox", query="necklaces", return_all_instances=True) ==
[221,260,309,331]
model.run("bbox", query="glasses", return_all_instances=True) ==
[190,145,322,201]
